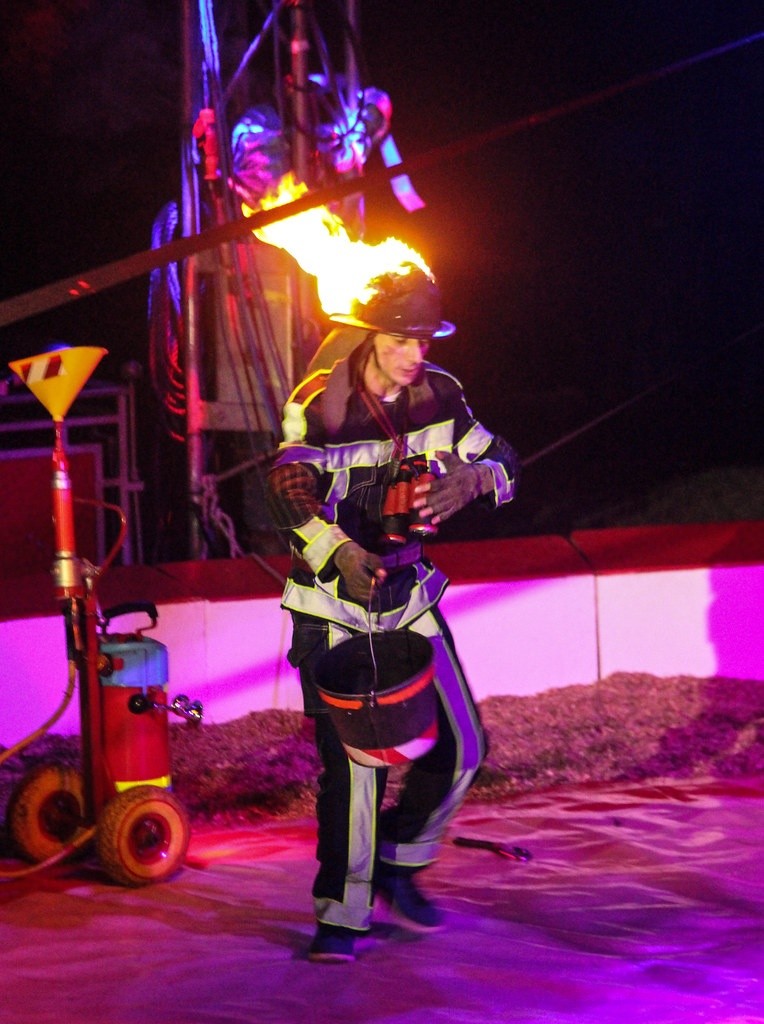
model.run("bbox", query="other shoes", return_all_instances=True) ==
[373,858,439,931]
[310,913,356,964]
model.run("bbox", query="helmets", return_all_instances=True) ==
[327,254,457,341]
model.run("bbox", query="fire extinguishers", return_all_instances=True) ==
[93,600,173,794]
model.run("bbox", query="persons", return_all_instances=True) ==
[264,243,519,963]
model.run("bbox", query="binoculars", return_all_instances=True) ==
[380,457,440,546]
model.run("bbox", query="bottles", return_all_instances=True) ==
[377,461,439,545]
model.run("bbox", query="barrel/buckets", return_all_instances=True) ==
[311,578,438,768]
